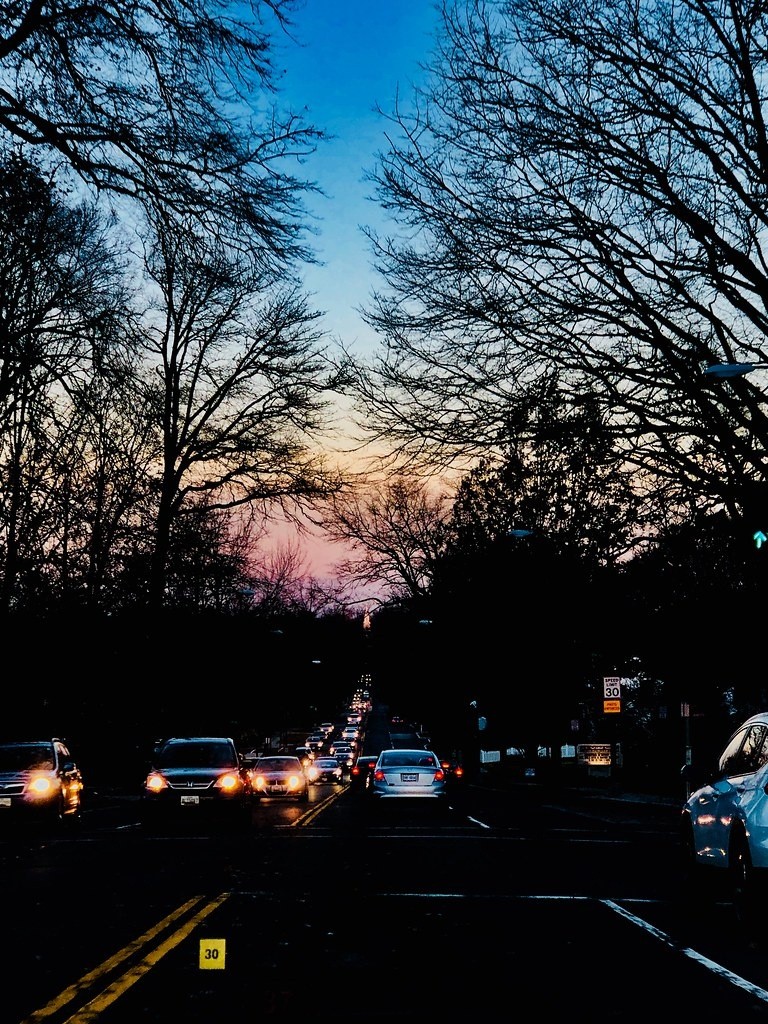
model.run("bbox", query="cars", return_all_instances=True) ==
[372,749,447,811]
[294,747,314,765]
[305,722,334,751]
[328,673,373,768]
[239,757,259,780]
[252,756,309,804]
[351,755,379,789]
[309,757,342,786]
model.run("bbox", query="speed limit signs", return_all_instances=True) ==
[603,676,622,698]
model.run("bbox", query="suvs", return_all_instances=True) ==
[0,737,84,840]
[143,737,247,828]
[679,712,768,899]
